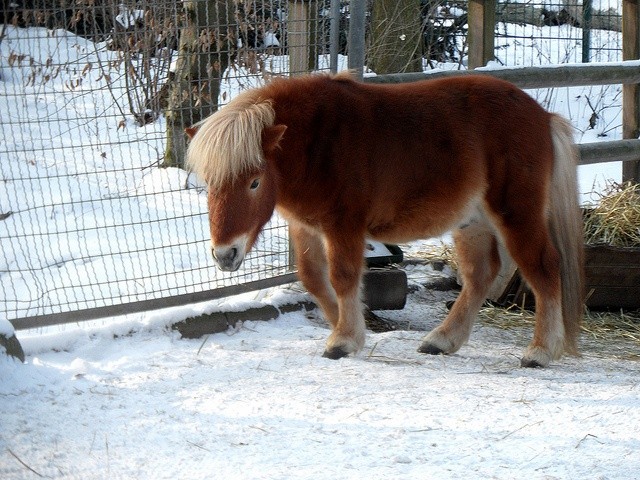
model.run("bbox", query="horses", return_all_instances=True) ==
[184,66,585,369]
